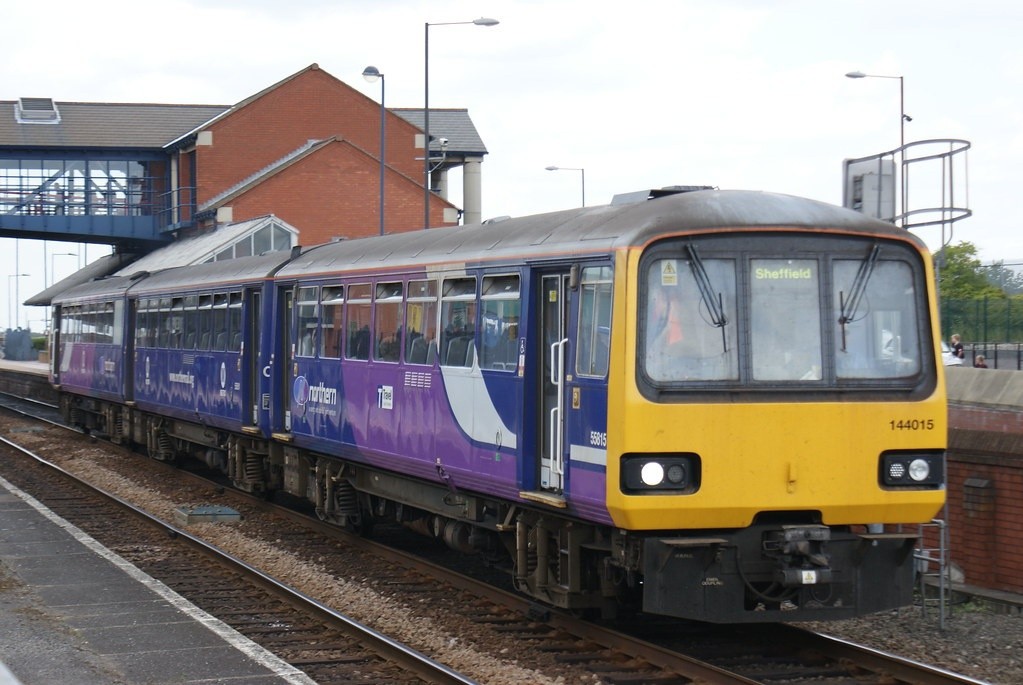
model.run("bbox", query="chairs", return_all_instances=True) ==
[345,327,523,372]
[135,327,243,353]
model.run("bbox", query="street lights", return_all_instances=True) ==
[50,251,80,285]
[6,272,30,332]
[845,71,909,231]
[421,16,502,232]
[362,64,389,234]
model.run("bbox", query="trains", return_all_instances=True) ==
[48,189,949,626]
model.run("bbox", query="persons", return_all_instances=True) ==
[951,333,966,358]
[55,183,65,215]
[975,355,987,368]
